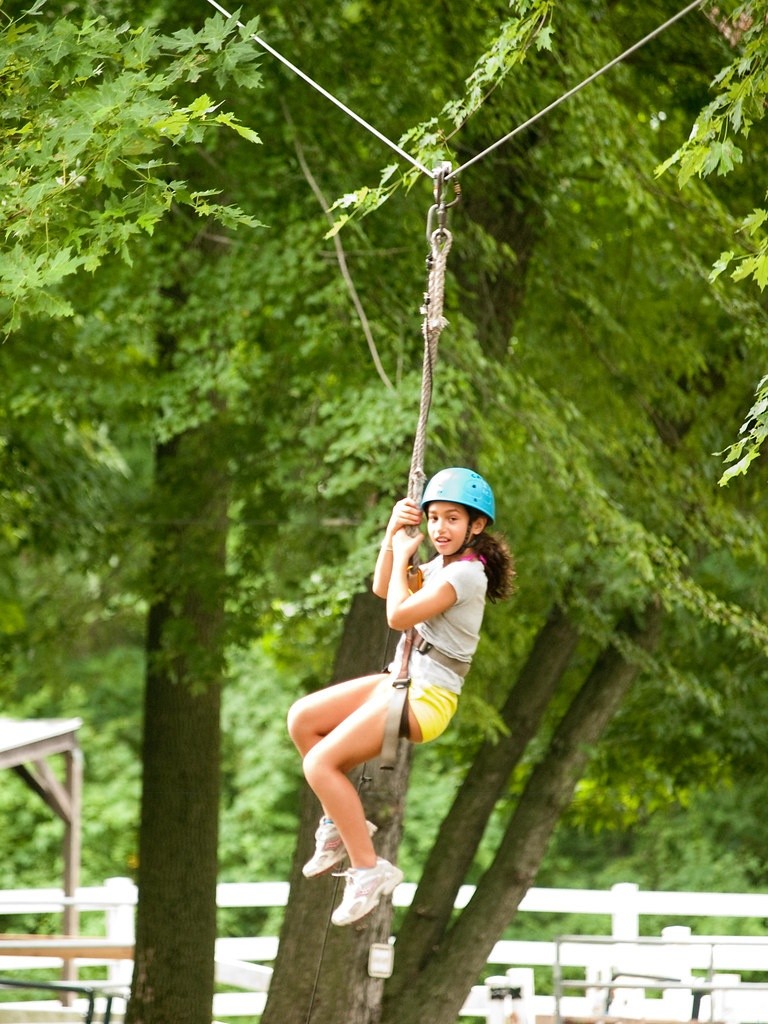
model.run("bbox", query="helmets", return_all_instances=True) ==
[420,468,495,526]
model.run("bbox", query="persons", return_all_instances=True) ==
[286,466,516,926]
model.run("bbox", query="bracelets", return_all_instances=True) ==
[380,543,392,551]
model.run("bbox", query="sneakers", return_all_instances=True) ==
[326,854,403,927]
[302,815,378,880]
